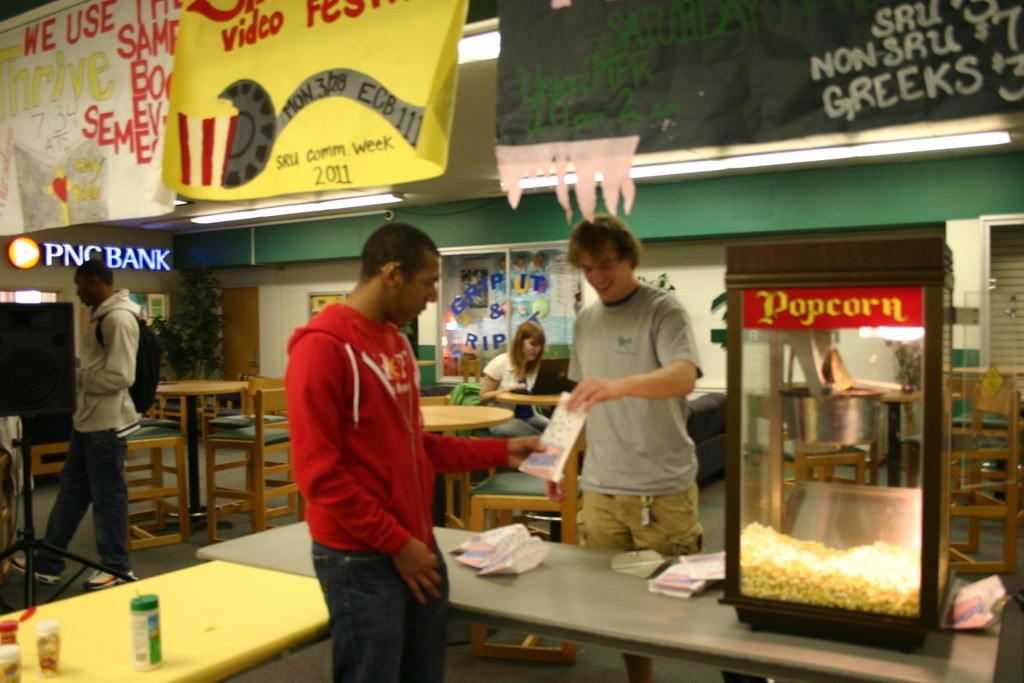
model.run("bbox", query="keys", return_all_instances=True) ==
[649,510,661,529]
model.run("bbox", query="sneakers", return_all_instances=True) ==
[12,544,136,593]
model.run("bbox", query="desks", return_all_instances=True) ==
[0,561,333,683]
[421,405,514,531]
[495,392,562,407]
[198,521,1003,683]
[153,381,249,519]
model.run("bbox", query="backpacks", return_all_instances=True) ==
[95,306,173,417]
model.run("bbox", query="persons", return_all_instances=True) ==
[286,222,547,683]
[479,320,551,437]
[546,213,715,683]
[8,258,162,589]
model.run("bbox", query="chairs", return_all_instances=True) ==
[33,377,583,549]
[950,379,1020,576]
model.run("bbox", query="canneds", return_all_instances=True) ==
[129,593,162,670]
